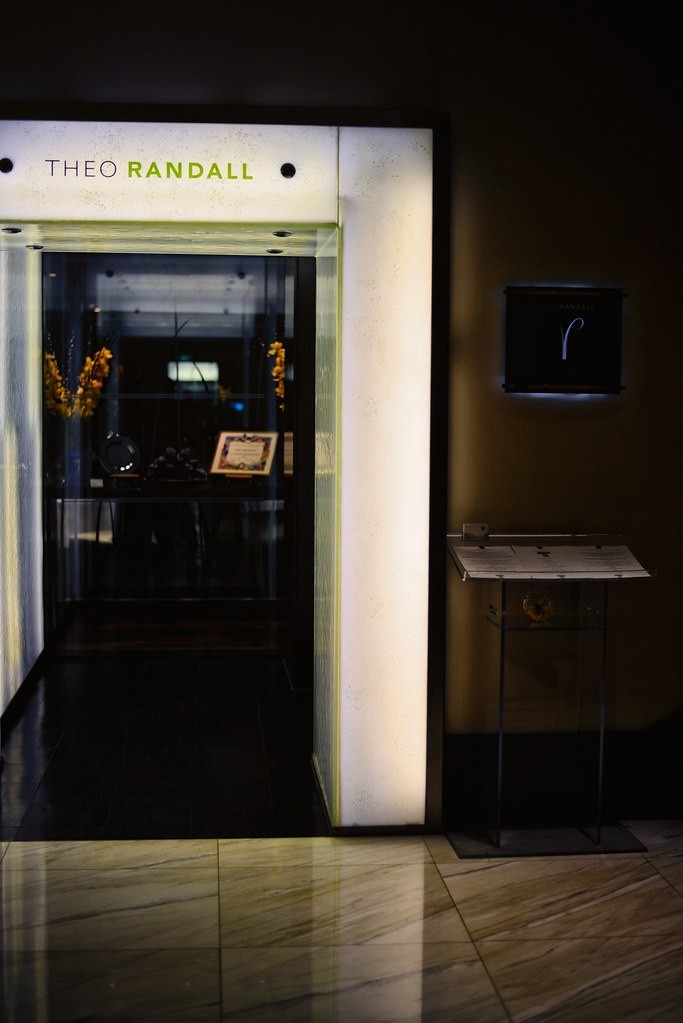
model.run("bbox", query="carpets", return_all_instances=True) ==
[444,810,650,860]
[53,619,289,657]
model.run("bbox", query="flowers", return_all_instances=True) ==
[264,340,284,413]
[43,325,115,419]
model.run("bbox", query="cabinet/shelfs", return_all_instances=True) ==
[57,488,278,619]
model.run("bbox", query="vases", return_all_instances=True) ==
[62,415,94,499]
